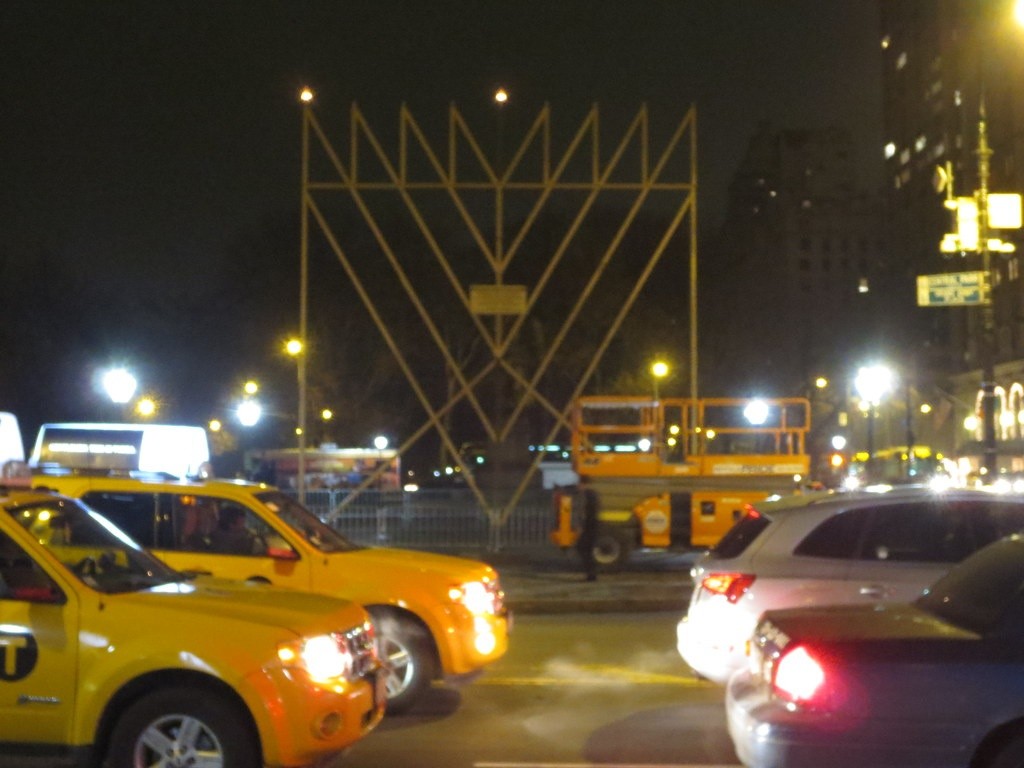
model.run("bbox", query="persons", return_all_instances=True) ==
[562,467,603,584]
[211,503,255,555]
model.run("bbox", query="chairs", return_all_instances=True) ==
[176,504,198,545]
[199,504,219,540]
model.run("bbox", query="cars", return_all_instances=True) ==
[724,534,1024,768]
[0,412,388,768]
[673,484,1024,684]
[27,423,514,718]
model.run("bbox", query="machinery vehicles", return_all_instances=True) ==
[547,396,811,574]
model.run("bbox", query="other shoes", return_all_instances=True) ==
[583,574,597,581]
